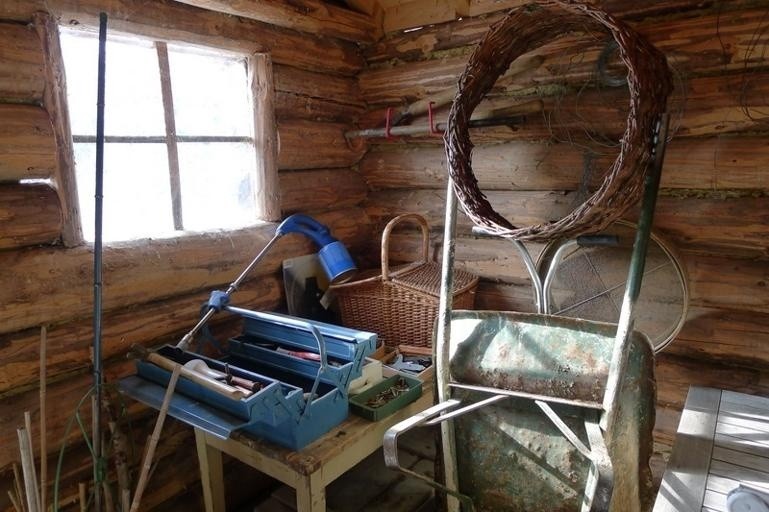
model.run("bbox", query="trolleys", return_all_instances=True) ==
[383,110,672,512]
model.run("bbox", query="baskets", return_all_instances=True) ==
[328,212,480,357]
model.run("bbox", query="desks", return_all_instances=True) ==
[651,385,769,512]
[191,346,445,512]
